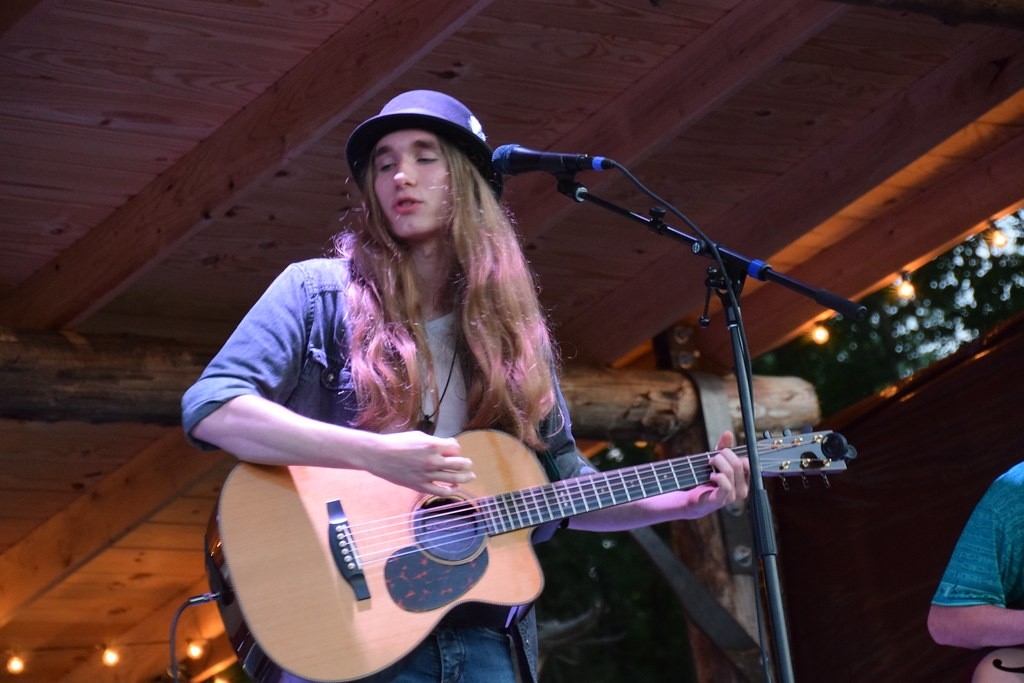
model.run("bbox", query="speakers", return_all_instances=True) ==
[653,322,702,372]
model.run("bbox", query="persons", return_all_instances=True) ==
[179,84,761,683]
[928,459,1024,682]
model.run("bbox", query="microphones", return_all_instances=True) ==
[492,144,612,175]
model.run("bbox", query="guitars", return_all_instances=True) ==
[205,426,850,683]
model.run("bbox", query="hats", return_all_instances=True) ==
[345,91,503,204]
